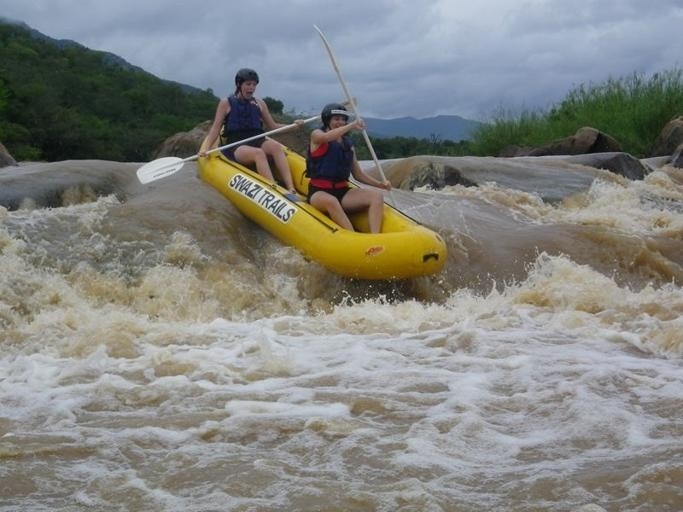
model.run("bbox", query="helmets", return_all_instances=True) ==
[322,103,349,129]
[235,68,259,92]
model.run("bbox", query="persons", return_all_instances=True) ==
[198,67,306,195]
[302,103,393,233]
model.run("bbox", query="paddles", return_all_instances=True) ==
[136,99,355,184]
[314,26,397,209]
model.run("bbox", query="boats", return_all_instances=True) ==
[197,123,448,280]
